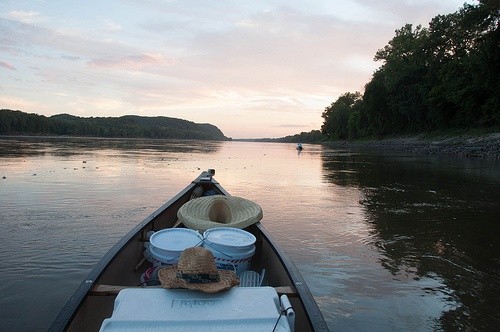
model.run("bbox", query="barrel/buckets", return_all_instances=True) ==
[150,227,202,269]
[203,228,257,275]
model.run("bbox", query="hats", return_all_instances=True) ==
[177,195,264,233]
[160,249,239,293]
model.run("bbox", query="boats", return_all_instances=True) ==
[296,143,303,150]
[46,169,330,331]
